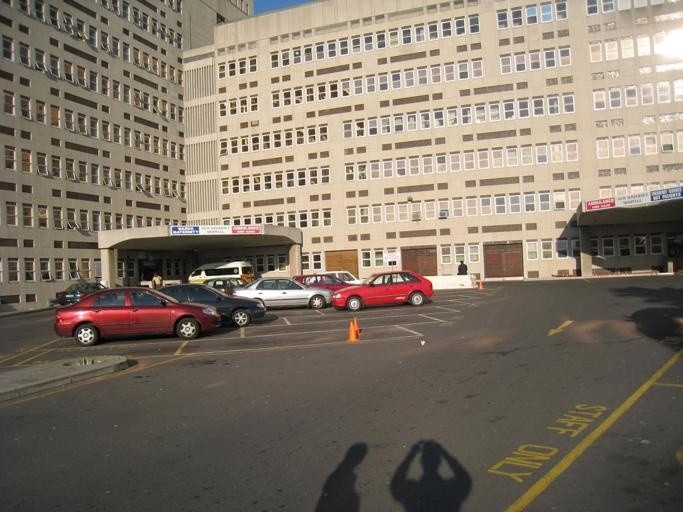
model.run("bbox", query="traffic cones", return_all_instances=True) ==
[353,317,359,336]
[348,320,356,342]
[479,280,483,290]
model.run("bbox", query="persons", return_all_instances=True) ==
[457,261,468,275]
[140,270,152,281]
[152,271,162,288]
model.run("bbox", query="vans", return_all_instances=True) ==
[187,261,254,283]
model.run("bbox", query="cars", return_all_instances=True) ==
[203,277,247,296]
[332,269,432,311]
[156,283,266,327]
[230,276,331,310]
[53,286,221,347]
[287,274,355,292]
[306,270,367,285]
[55,282,106,305]
